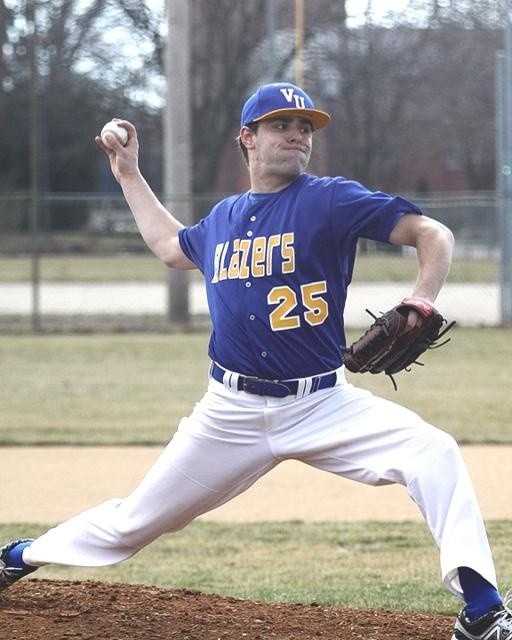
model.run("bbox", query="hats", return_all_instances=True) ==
[240,81,331,130]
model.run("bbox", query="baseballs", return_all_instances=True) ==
[101,121,129,149]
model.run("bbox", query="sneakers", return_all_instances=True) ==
[450,603,512,640]
[0,538,39,591]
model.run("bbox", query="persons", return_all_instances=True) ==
[0,81,512,640]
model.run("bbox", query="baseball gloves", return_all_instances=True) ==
[342,297,456,375]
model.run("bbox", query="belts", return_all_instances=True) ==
[210,362,337,398]
